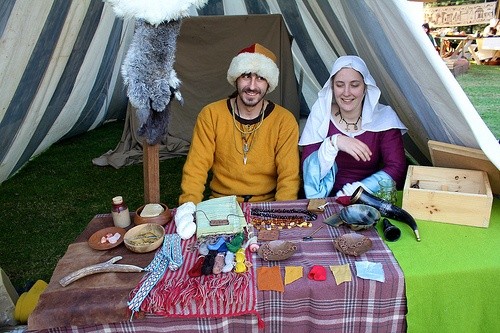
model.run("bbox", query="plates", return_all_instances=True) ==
[88,226,127,251]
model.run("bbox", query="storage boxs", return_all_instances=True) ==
[402,139,500,228]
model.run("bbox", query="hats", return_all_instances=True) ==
[226,41,281,95]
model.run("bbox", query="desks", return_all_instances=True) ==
[27,191,500,333]
[481,37,500,65]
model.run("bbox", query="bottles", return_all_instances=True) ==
[375,178,398,206]
[110,196,132,228]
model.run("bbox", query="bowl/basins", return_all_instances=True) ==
[123,223,166,253]
[338,203,381,231]
[132,203,171,224]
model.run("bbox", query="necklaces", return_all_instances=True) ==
[335,108,362,131]
[232,96,264,165]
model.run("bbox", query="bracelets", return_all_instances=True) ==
[330,136,337,151]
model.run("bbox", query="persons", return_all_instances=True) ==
[179,42,299,206]
[421,23,434,45]
[298,55,408,204]
